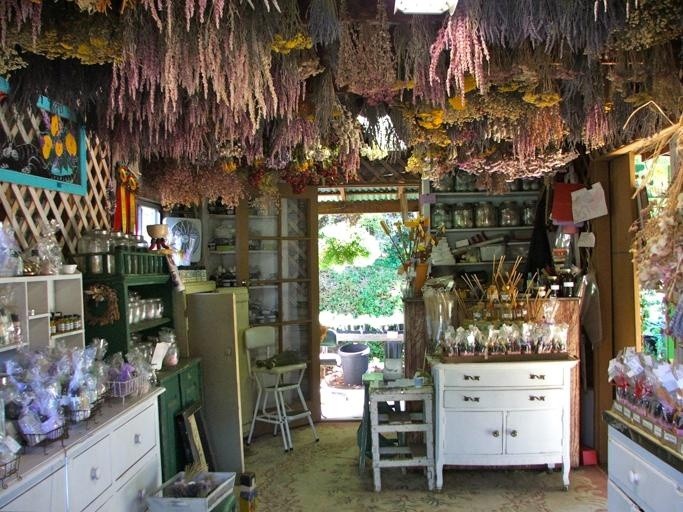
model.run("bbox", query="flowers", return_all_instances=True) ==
[0,0,683,219]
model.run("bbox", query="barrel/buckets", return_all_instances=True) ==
[338,344,370,385]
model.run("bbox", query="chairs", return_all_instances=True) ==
[244,326,320,453]
[319,329,349,401]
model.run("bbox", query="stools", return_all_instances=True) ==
[359,373,408,476]
[368,379,436,492]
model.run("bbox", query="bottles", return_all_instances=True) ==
[520,199,538,225]
[125,298,165,325]
[50,314,81,336]
[88,229,148,275]
[129,334,180,368]
[498,200,521,226]
[8,250,41,277]
[474,201,497,227]
[453,201,474,228]
[534,267,576,297]
[431,202,453,229]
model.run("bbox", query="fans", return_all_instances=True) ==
[161,218,202,263]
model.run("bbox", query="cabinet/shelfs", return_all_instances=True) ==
[422,177,540,279]
[606,424,683,512]
[68,251,204,482]
[201,197,289,327]
[0,269,167,512]
[434,361,577,494]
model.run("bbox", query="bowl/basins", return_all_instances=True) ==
[63,264,78,274]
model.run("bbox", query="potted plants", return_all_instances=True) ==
[174,462,208,497]
